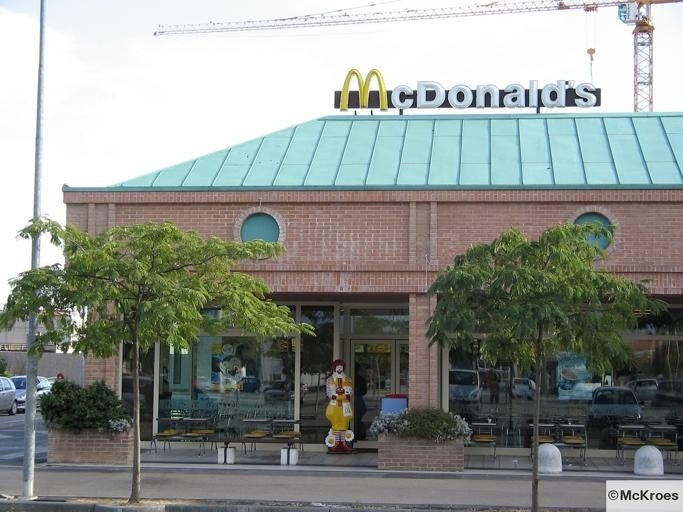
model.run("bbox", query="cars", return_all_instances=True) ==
[589,378,683,419]
[196,376,307,404]
[0,375,61,415]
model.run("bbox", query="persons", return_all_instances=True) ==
[484,364,501,405]
[323,360,357,453]
[56,373,64,380]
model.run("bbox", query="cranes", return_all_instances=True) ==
[151,0,683,113]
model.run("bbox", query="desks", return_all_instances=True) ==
[473,423,679,466]
[156,417,302,456]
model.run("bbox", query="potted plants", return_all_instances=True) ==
[36,378,136,466]
[369,405,472,472]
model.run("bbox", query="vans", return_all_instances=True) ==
[449,369,536,414]
